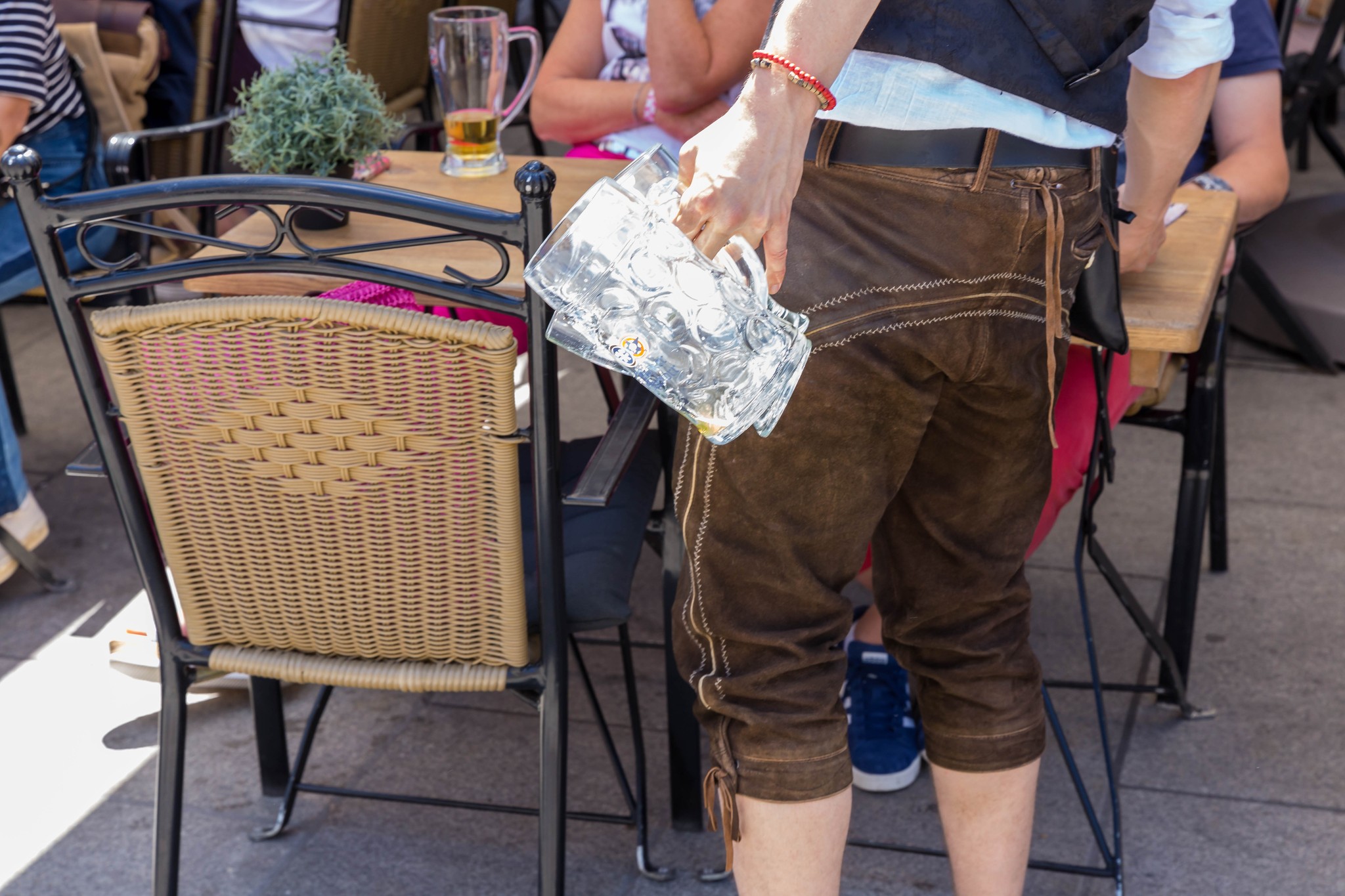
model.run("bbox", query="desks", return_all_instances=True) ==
[183,150,1243,720]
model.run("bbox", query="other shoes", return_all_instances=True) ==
[0,491,53,586]
[843,620,926,791]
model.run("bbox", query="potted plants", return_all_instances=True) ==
[226,38,408,232]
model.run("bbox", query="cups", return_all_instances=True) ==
[427,5,544,177]
[522,144,815,446]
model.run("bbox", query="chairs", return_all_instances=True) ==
[0,0,1345,896]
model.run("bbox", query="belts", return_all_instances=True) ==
[803,119,1097,171]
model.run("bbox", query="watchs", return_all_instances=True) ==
[1182,174,1235,192]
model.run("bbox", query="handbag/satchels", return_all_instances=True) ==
[1068,144,1129,354]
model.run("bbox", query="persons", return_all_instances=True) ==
[0,0,105,582]
[840,0,1290,795]
[107,0,779,688]
[671,0,1237,896]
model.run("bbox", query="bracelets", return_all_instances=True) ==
[644,85,659,122]
[750,48,837,111]
[634,81,643,124]
